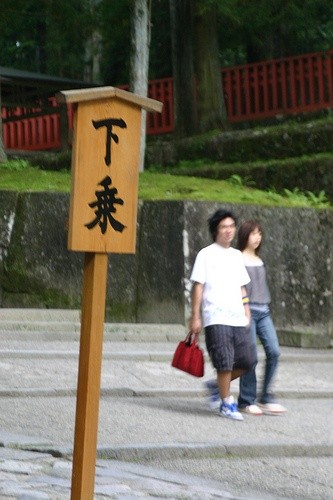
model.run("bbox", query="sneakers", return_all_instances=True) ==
[220,399,243,420]
[205,382,224,411]
[242,400,286,414]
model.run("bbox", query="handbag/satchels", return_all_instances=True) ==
[171,341,205,377]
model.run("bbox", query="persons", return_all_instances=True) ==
[189,209,258,421]
[236,221,288,415]
